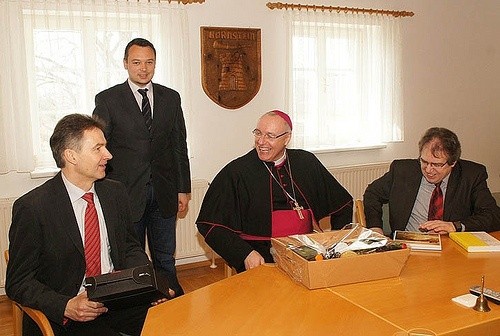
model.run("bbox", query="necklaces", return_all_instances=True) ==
[263,154,304,220]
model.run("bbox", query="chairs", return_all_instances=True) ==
[4,249,55,336]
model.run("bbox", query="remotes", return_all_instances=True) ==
[470,285,500,303]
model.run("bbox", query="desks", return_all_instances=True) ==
[140,229,500,336]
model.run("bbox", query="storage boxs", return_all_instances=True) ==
[269,227,411,291]
[84,265,169,309]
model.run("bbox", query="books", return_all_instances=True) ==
[394,230,442,250]
[449,231,500,253]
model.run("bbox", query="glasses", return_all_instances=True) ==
[252,128,289,141]
[418,155,448,168]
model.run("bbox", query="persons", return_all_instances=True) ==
[5,113,175,336]
[363,127,500,236]
[93,38,191,298]
[195,110,354,273]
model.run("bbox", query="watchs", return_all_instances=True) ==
[453,221,462,232]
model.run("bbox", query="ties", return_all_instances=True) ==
[81,193,101,278]
[428,180,443,221]
[137,88,153,133]
